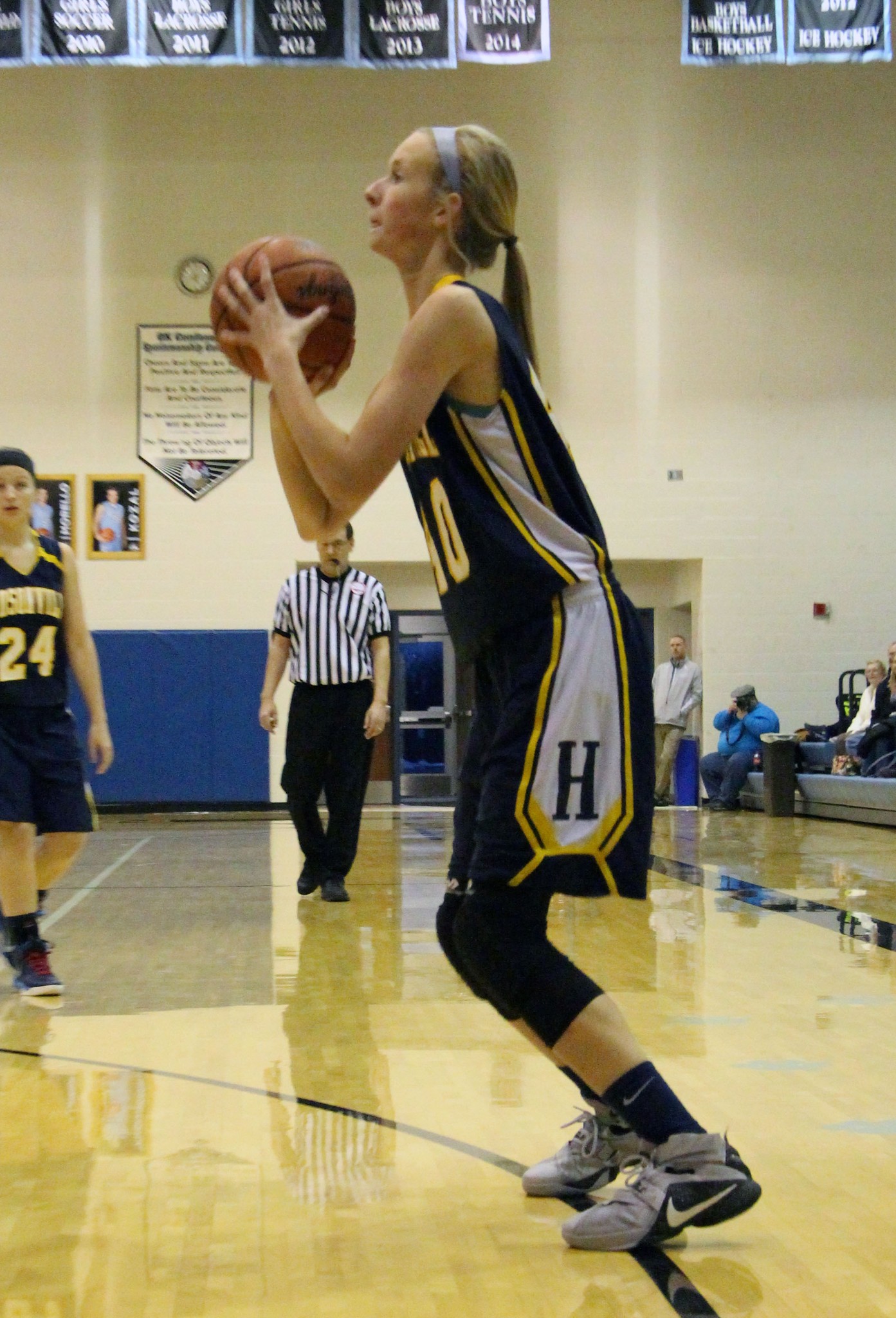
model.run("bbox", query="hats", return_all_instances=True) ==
[731,685,755,695]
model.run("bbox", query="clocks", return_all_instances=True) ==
[175,255,216,298]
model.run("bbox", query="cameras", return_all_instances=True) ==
[736,699,749,708]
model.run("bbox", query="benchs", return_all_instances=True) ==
[736,740,896,828]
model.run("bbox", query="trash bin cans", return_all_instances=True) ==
[761,734,798,817]
[673,733,700,806]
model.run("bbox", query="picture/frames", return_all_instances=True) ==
[86,474,143,560]
[30,473,76,554]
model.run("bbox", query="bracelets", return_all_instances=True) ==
[259,692,273,699]
[373,695,388,704]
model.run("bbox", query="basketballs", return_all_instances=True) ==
[210,236,356,385]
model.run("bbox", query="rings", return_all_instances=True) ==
[376,725,383,731]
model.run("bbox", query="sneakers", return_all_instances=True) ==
[520,1094,655,1197]
[320,873,347,905]
[564,1131,761,1252]
[4,931,65,997]
[297,854,321,894]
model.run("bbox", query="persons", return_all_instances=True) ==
[31,489,55,533]
[259,521,392,904]
[218,125,766,1254]
[699,685,780,811]
[654,635,702,808]
[793,642,896,779]
[0,446,115,996]
[92,488,127,552]
[264,903,398,1257]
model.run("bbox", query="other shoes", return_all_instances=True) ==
[654,797,668,806]
[706,800,732,812]
